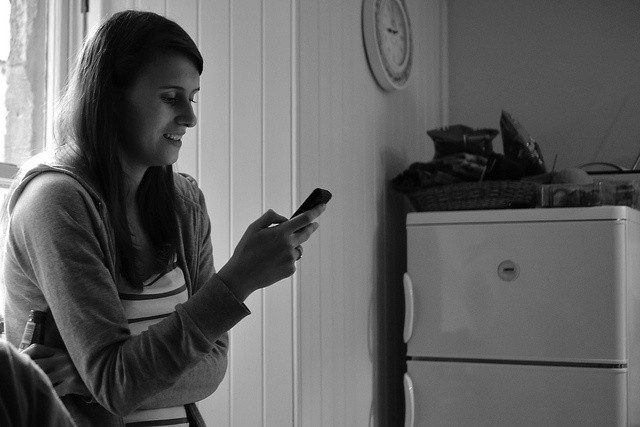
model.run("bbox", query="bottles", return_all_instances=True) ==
[14,309,46,349]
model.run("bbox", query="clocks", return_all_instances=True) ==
[360,0,418,93]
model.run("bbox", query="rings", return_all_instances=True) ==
[295,248,303,261]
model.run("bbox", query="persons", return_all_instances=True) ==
[0,9,328,427]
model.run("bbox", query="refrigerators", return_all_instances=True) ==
[402,205,640,427]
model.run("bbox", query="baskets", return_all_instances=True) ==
[409,181,540,213]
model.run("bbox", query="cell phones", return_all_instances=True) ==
[291,188,332,218]
